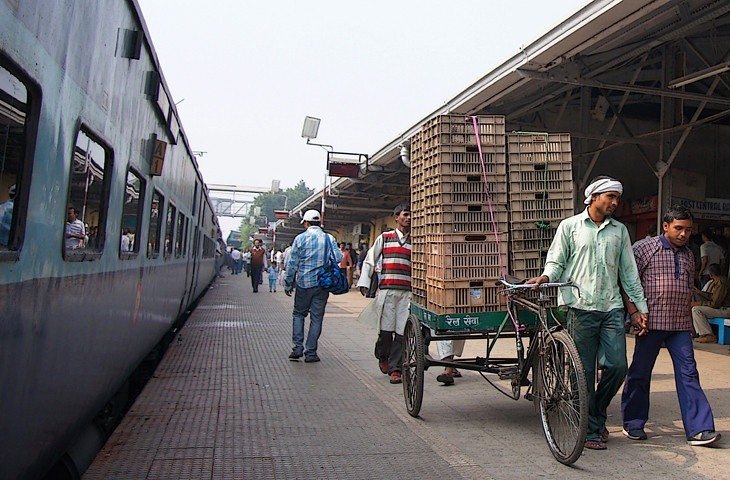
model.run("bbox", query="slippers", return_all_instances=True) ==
[584,437,607,450]
[599,426,609,442]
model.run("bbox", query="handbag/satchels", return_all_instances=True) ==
[317,232,349,295]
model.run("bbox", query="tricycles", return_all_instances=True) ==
[400,280,589,464]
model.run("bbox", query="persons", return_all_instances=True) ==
[282,209,343,362]
[688,228,730,343]
[524,176,650,449]
[618,205,721,446]
[357,204,414,383]
[1,184,182,253]
[436,339,466,385]
[216,238,368,292]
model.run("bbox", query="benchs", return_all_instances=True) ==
[707,318,730,344]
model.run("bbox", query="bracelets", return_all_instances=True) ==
[629,309,639,317]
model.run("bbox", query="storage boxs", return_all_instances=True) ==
[411,114,574,313]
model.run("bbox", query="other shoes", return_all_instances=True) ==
[305,355,320,363]
[694,336,717,344]
[450,371,462,377]
[288,351,303,358]
[437,373,454,383]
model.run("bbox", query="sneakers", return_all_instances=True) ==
[622,429,647,440]
[687,431,722,445]
[379,359,389,374]
[390,372,402,383]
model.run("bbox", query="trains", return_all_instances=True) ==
[0,0,228,480]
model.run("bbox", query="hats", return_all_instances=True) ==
[8,184,16,194]
[300,209,321,225]
